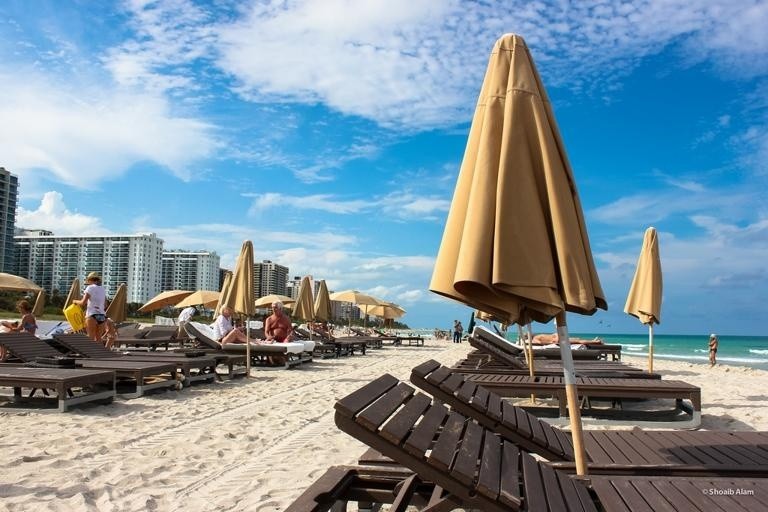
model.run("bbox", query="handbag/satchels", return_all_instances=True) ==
[63,303,87,331]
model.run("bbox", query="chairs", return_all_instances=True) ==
[0,323,768,510]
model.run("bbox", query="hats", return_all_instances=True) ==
[86,271,102,282]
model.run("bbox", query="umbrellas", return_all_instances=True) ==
[62,279,81,312]
[106,283,127,322]
[624,226,664,374]
[0,272,41,290]
[32,287,45,317]
[138,240,407,378]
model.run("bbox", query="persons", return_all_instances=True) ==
[0,300,38,361]
[458,322,463,343]
[524,333,602,345]
[73,271,120,350]
[709,333,718,367]
[453,320,460,343]
